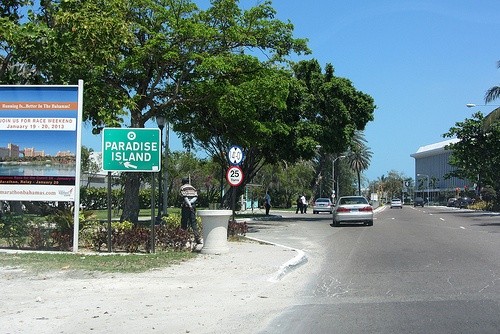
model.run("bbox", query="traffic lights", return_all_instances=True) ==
[456,187,460,196]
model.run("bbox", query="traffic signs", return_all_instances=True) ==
[102,127,161,171]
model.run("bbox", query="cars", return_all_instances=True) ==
[446,197,475,209]
[333,196,373,227]
[414,197,424,207]
[313,198,334,214]
[390,199,402,208]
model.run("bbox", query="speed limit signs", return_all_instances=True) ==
[226,166,244,185]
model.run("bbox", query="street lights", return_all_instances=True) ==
[220,150,226,210]
[318,175,322,197]
[417,173,429,206]
[332,156,345,204]
[156,113,165,225]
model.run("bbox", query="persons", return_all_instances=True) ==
[179,178,201,240]
[301,193,307,214]
[296,195,304,214]
[265,191,272,216]
